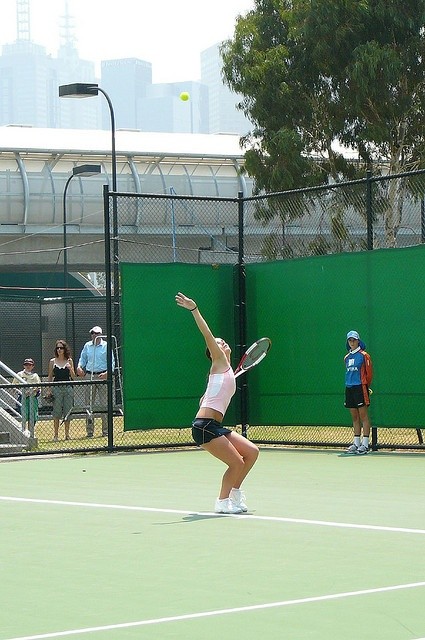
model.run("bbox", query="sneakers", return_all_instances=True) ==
[355,445,370,454]
[344,444,360,453]
[229,488,247,512]
[214,498,242,513]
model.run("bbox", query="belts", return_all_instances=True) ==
[86,369,107,375]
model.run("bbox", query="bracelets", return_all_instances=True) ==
[189,305,197,312]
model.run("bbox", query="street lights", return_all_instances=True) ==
[58,83,120,405]
[63,165,101,346]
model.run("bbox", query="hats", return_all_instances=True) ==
[346,330,359,339]
[23,359,34,365]
[89,327,102,334]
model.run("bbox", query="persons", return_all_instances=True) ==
[172,291,255,515]
[340,328,373,454]
[45,339,76,440]
[75,324,117,438]
[9,357,42,439]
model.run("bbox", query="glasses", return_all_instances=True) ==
[56,347,65,349]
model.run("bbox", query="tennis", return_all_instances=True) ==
[180,92,189,101]
